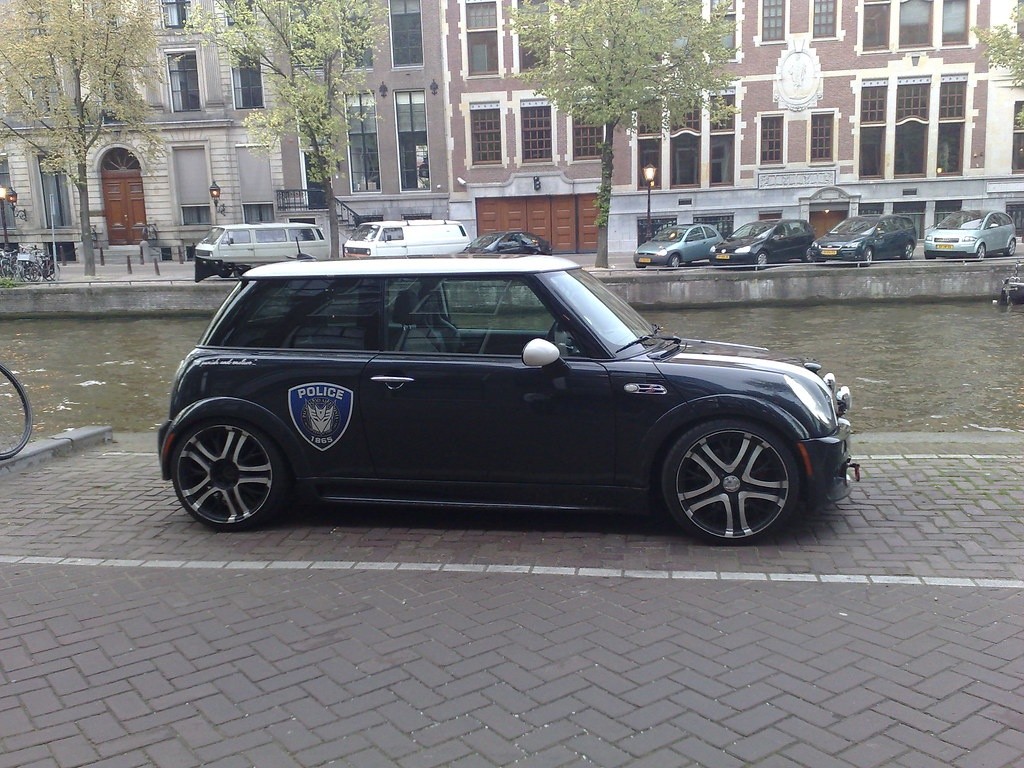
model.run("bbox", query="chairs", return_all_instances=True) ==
[792,227,802,233]
[389,290,461,352]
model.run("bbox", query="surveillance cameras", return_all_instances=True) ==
[457,177,466,186]
[973,154,977,158]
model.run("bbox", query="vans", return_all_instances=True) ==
[195,222,331,282]
[343,218,470,256]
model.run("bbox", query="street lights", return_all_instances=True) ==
[0,185,13,251]
[642,163,657,241]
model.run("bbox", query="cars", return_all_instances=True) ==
[922,209,1017,262]
[634,222,726,268]
[808,215,918,267]
[155,256,861,547]
[460,231,554,257]
[708,218,818,270]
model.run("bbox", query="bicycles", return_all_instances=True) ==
[0,245,60,284]
[0,363,35,460]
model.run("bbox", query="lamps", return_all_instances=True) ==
[6,187,27,222]
[209,181,225,216]
[534,176,540,190]
